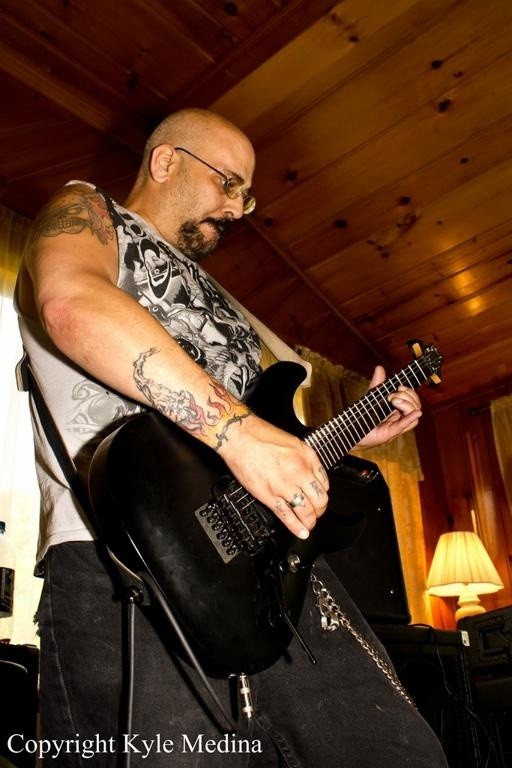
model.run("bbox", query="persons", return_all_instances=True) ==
[11,107,449,767]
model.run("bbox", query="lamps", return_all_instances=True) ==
[426,532,504,630]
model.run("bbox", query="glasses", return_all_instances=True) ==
[176,147,256,215]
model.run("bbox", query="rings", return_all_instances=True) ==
[289,495,304,507]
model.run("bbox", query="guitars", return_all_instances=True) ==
[73,342,439,674]
